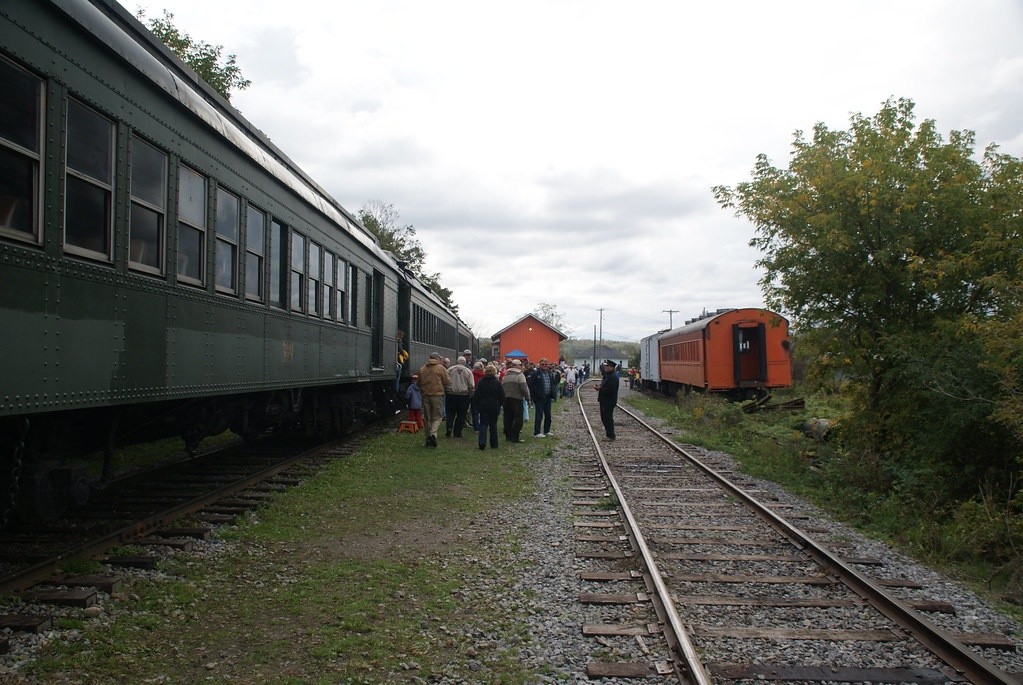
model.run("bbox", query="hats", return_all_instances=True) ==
[430,352,443,359]
[464,350,472,355]
[479,358,487,363]
[411,375,419,382]
[603,359,617,368]
[511,359,524,365]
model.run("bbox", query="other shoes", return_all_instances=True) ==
[454,434,464,437]
[475,429,481,434]
[428,433,437,448]
[445,428,452,436]
[602,437,615,442]
[512,439,524,443]
[425,440,431,447]
[534,433,546,438]
[479,443,485,450]
[544,432,554,436]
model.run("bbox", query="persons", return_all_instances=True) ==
[441,358,450,421]
[600,359,622,379]
[445,349,561,450]
[560,359,590,399]
[393,329,403,394]
[593,360,619,441]
[418,352,452,448]
[404,372,424,430]
[627,366,643,391]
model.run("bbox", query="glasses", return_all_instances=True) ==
[540,364,549,366]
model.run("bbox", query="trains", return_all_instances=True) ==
[0,0,477,437]
[640,308,793,405]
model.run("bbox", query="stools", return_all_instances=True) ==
[397,420,419,435]
